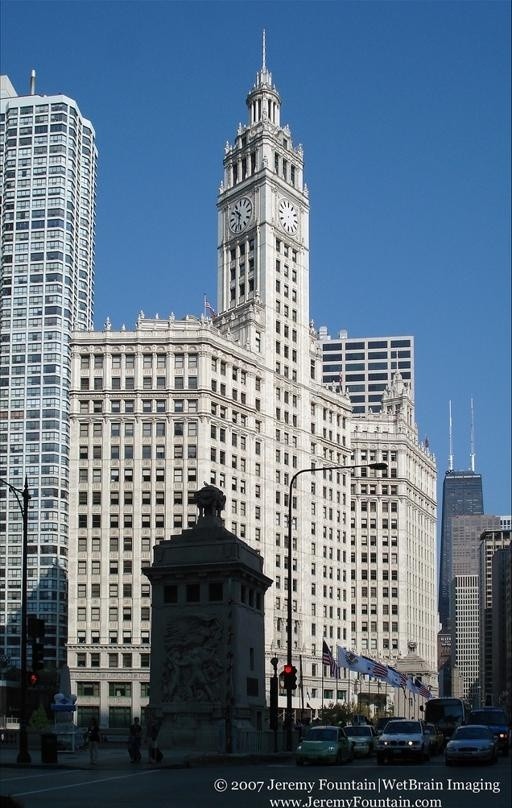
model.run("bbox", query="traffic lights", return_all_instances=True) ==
[291,667,298,691]
[283,665,294,690]
[25,672,43,692]
[30,643,46,671]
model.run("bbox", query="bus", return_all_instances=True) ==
[419,696,471,741]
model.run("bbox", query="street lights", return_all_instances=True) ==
[284,462,391,745]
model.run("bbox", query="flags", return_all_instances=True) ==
[321,640,435,702]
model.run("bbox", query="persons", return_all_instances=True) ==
[87,716,101,765]
[127,716,142,763]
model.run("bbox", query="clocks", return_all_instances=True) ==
[230,197,251,233]
[279,200,297,233]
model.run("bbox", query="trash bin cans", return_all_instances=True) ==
[40,732,58,764]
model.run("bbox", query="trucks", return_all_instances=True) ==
[469,704,511,759]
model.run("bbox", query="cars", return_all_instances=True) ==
[443,724,500,766]
[421,722,445,755]
[296,725,352,767]
[342,724,378,758]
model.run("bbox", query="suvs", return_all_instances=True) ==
[374,719,431,766]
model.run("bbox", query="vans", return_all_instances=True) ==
[373,716,408,751]
